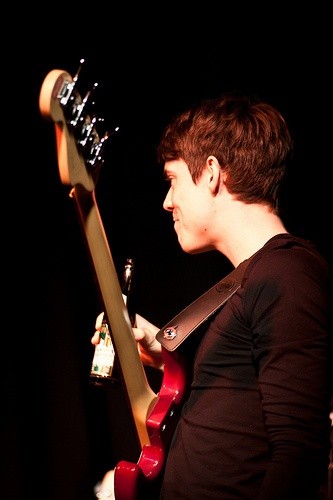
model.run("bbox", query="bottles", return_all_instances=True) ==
[88,259,134,386]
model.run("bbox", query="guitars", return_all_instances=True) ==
[40,58,192,500]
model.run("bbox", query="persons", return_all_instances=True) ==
[91,93,333,500]
